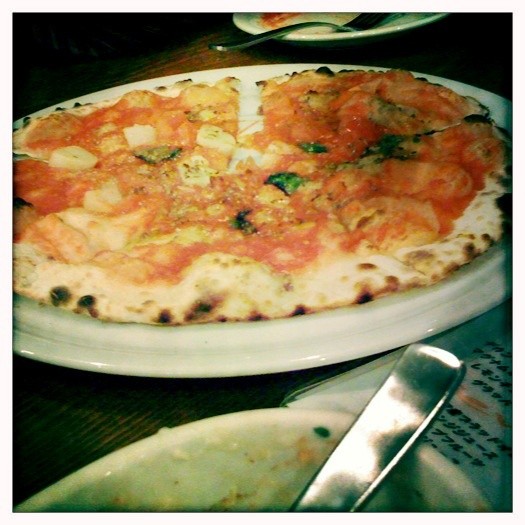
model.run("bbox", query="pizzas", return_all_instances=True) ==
[255,15,357,32]
[14,66,510,326]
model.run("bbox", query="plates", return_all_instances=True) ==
[12,60,512,376]
[226,12,450,52]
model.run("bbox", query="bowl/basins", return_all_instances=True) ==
[15,407,499,512]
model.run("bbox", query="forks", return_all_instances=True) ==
[207,12,394,52]
